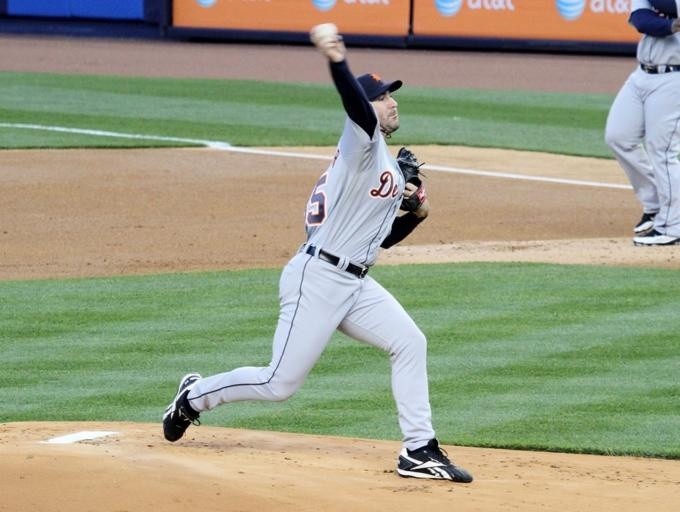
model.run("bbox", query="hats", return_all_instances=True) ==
[358,73,402,101]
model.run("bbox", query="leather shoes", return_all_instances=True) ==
[308,246,368,279]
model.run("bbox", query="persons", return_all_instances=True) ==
[602,0,679,246]
[161,22,474,484]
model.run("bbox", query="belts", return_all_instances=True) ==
[640,64,676,73]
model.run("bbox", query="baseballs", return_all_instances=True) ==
[314,24,338,43]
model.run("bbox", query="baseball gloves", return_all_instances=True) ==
[395,148,426,210]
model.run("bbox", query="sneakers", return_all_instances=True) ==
[633,214,657,233]
[633,229,678,246]
[396,438,473,483]
[164,374,203,442]
